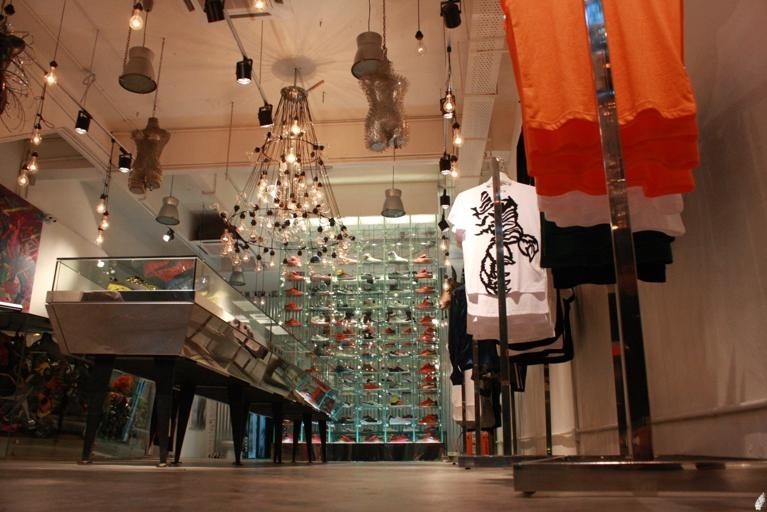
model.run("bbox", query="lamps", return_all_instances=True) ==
[219,67,356,275]
[95,138,115,245]
[74,28,99,135]
[201,1,225,23]
[234,0,273,128]
[119,1,180,243]
[350,0,465,235]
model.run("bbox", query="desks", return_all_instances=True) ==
[1,302,78,432]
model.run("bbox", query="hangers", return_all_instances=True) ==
[486,154,512,186]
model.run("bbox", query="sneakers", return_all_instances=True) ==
[418,315,436,323]
[418,414,439,425]
[412,254,433,264]
[416,298,434,308]
[416,349,437,357]
[415,286,435,294]
[421,375,437,383]
[420,327,436,342]
[415,363,436,374]
[283,252,417,443]
[414,268,433,279]
[416,397,438,407]
[416,382,437,390]
